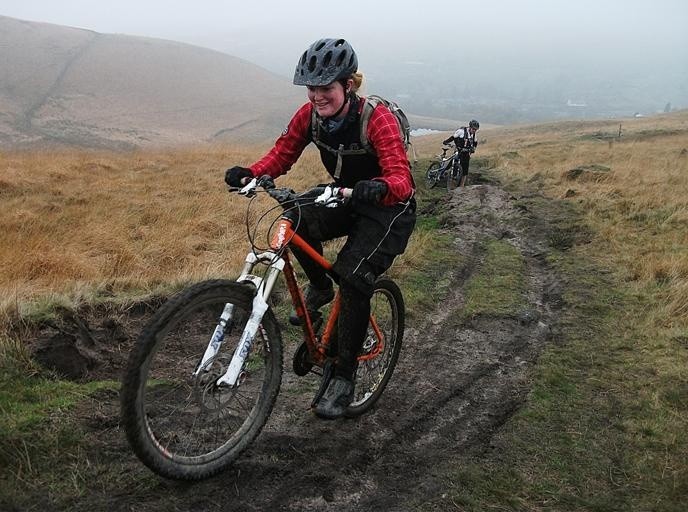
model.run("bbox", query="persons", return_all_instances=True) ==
[224,38,418,425]
[442,119,480,190]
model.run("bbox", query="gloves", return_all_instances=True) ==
[353,179,389,203]
[224,165,255,189]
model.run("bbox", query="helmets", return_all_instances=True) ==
[292,38,358,89]
[469,120,479,130]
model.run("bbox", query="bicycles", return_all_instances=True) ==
[115,171,408,482]
[423,139,474,192]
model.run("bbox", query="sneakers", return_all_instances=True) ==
[314,377,355,419]
[289,276,335,327]
